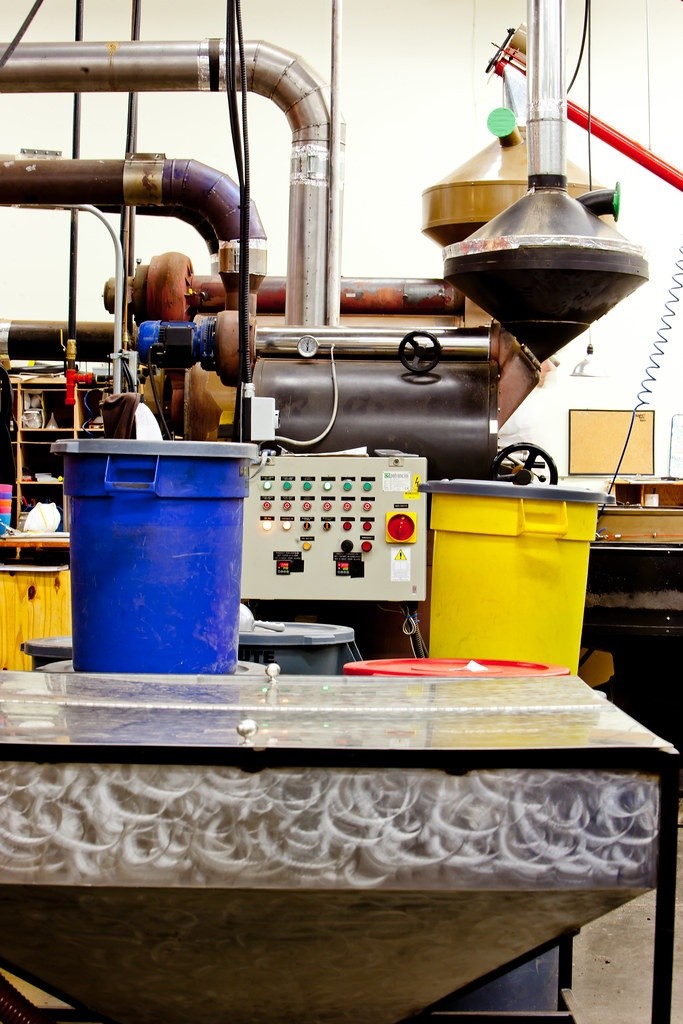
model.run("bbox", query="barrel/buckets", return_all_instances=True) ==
[340,656,570,1024]
[24,636,74,670]
[417,479,616,675]
[48,439,259,674]
[238,620,363,674]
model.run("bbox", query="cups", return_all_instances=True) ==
[0,483,13,535]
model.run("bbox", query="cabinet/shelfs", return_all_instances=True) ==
[1,376,113,538]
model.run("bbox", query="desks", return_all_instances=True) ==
[1,671,683,1024]
